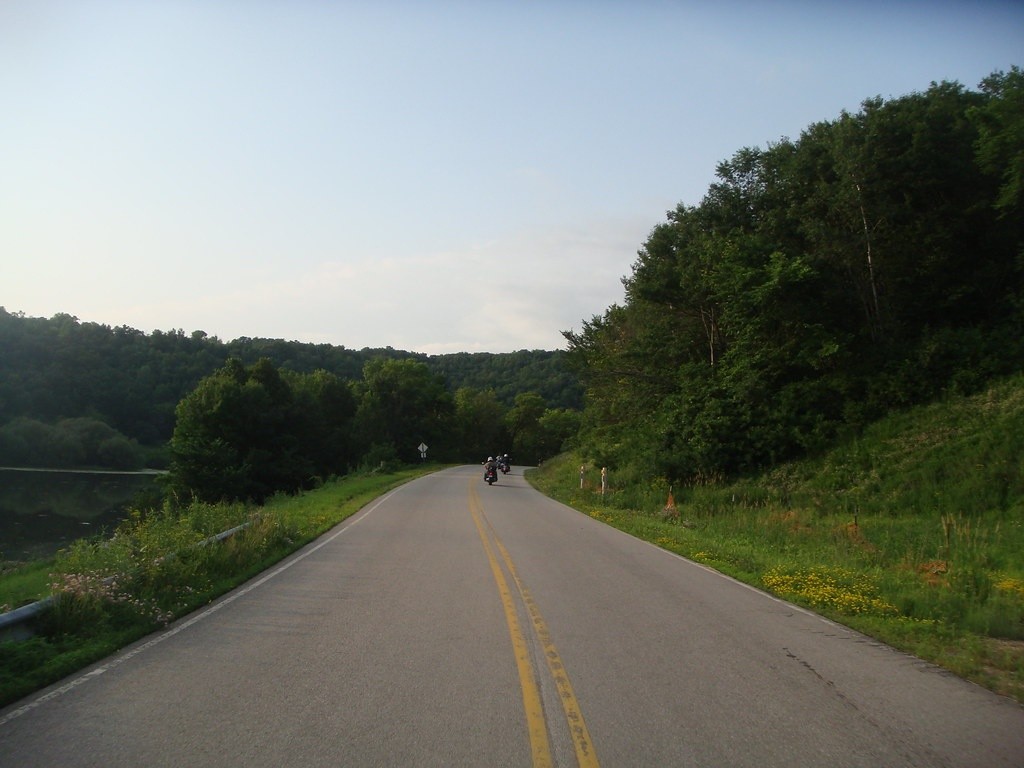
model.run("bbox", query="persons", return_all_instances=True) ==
[484,456,497,481]
[496,453,510,471]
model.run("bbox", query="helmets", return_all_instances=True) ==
[488,457,493,461]
[504,454,508,457]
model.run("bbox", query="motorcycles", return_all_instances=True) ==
[482,462,500,485]
[499,458,512,475]
